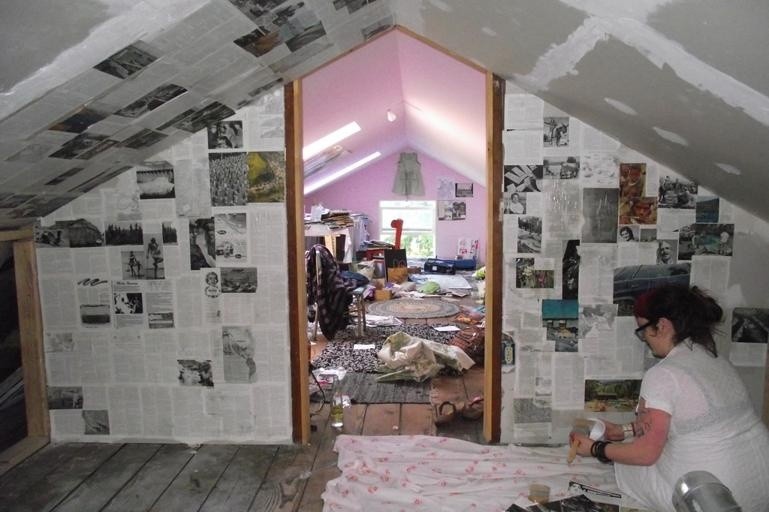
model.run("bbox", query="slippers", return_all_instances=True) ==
[463,398,483,421]
[434,401,466,428]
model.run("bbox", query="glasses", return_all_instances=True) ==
[634,322,653,342]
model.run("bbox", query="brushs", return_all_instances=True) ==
[527,484,551,505]
[567,419,597,463]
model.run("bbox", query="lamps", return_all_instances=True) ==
[387,109,396,122]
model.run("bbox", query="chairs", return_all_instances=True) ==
[312,252,367,339]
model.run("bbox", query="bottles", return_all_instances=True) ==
[330,375,344,427]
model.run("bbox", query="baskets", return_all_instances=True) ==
[387,259,408,284]
[374,289,393,300]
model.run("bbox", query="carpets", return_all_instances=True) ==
[369,299,460,319]
[409,274,478,291]
[311,367,432,403]
[311,323,468,373]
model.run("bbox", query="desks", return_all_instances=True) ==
[304,219,370,261]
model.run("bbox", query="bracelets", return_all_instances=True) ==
[621,422,636,441]
[591,441,614,463]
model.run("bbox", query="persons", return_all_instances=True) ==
[125,251,143,280]
[618,226,637,242]
[147,238,165,280]
[203,271,221,323]
[566,283,768,510]
[506,192,526,215]
[656,240,676,265]
